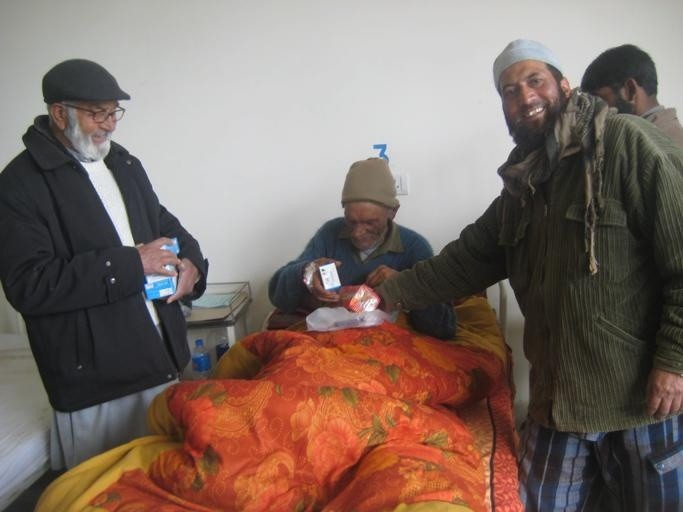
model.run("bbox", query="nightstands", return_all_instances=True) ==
[179,292,251,380]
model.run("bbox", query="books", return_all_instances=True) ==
[186,281,250,321]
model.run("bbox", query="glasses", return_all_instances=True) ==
[57,103,126,123]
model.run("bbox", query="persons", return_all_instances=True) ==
[352,40,683,512]
[579,44,683,162]
[2,58,208,471]
[268,158,456,343]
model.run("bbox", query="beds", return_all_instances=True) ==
[36,279,527,511]
[1,332,53,512]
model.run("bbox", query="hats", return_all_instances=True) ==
[492,38,565,88]
[41,58,131,104]
[340,157,399,208]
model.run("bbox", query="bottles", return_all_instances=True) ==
[216,336,229,363]
[191,339,212,380]
